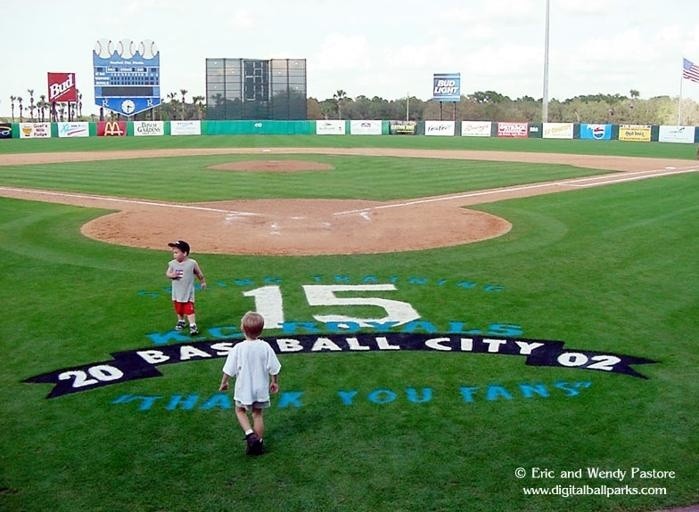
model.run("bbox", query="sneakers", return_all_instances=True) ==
[243,433,263,454]
[175,322,198,335]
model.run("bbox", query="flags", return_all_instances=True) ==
[683,59,699,82]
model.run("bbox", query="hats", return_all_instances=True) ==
[168,241,189,256]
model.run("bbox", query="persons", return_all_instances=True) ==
[166,241,206,335]
[219,311,282,455]
